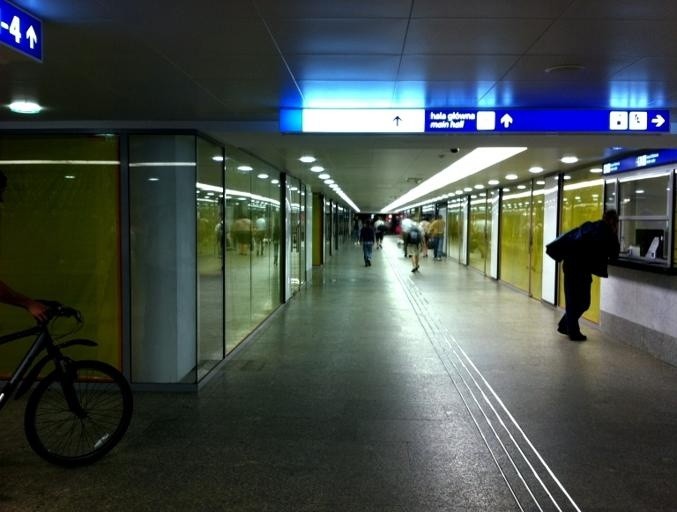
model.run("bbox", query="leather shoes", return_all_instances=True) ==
[557,325,586,342]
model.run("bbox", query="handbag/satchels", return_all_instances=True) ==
[546,227,578,262]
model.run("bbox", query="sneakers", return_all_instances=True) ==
[404,254,442,272]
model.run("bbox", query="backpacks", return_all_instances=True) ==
[408,230,419,244]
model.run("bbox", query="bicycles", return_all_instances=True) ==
[0,299,133,467]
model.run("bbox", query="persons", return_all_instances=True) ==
[199,207,306,259]
[1,281,50,322]
[558,209,624,340]
[351,213,448,272]
